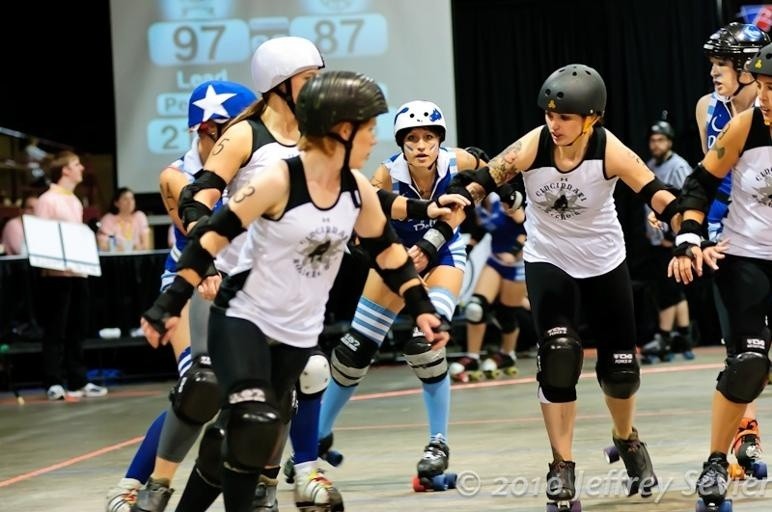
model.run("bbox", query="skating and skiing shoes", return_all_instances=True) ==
[547,460,581,512]
[132,487,174,512]
[604,427,658,497]
[285,433,342,484]
[413,433,457,491]
[449,353,484,383]
[252,475,279,512]
[731,417,767,479]
[295,469,344,512]
[641,324,694,364]
[696,452,733,512]
[105,478,149,512]
[481,350,518,379]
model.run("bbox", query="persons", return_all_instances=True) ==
[696,23,772,481]
[449,145,529,383]
[639,120,694,363]
[668,44,772,512]
[139,72,452,512]
[1,132,175,400]
[407,63,729,512]
[283,101,523,493]
[105,80,347,512]
[132,36,325,512]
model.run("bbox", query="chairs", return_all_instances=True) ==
[1,207,23,230]
[83,205,102,228]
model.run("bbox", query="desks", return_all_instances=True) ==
[149,213,172,226]
[1,248,176,342]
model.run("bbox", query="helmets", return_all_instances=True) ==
[251,36,325,93]
[704,22,771,73]
[394,101,447,146]
[749,44,772,80]
[652,121,674,139]
[295,71,388,136]
[189,80,257,133]
[537,64,608,117]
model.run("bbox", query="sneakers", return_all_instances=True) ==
[67,382,108,397]
[47,385,66,400]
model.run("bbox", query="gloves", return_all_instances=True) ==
[415,221,453,259]
[142,275,194,336]
[672,220,702,261]
[406,192,447,220]
[404,284,451,333]
